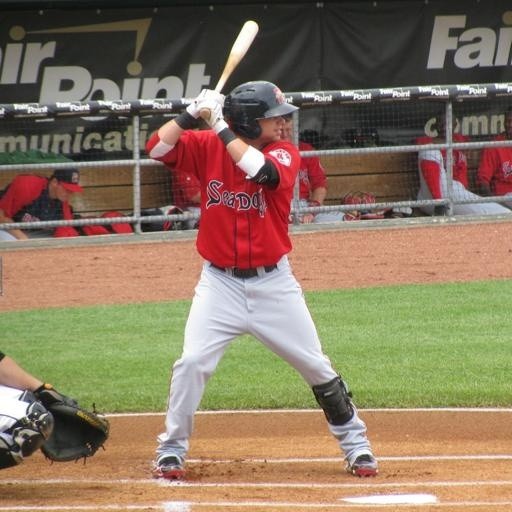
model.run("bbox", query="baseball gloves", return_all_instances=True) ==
[34,383,108,466]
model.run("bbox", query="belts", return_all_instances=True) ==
[209,262,277,278]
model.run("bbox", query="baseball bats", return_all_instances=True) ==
[200,20,259,120]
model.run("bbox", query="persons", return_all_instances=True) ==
[406,109,512,216]
[142,77,380,480]
[472,102,512,201]
[169,168,204,211]
[2,348,112,488]
[273,112,329,226]
[0,165,86,243]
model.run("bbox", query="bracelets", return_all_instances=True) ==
[175,110,196,130]
[219,128,236,146]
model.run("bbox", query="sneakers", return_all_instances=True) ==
[155,452,185,482]
[344,446,379,478]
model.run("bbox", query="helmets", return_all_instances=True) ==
[223,80,301,139]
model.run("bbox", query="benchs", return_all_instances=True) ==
[0,149,494,216]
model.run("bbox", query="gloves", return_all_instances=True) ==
[186,88,229,136]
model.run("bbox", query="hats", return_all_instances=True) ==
[52,166,85,193]
